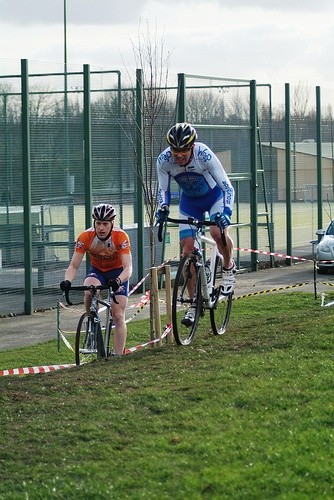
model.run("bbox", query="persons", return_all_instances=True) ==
[60,203,133,354]
[155,122,236,325]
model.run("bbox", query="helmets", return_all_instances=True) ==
[92,204,116,221]
[166,121,198,150]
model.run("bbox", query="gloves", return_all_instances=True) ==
[217,213,231,230]
[60,279,72,291]
[154,206,170,223]
[107,278,120,292]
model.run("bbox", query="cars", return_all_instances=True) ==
[314,218,334,273]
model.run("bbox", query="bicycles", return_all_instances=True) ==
[66,285,121,367]
[155,214,235,346]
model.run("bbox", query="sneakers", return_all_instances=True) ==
[220,258,238,296]
[181,301,198,324]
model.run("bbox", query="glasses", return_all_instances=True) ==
[170,145,193,154]
